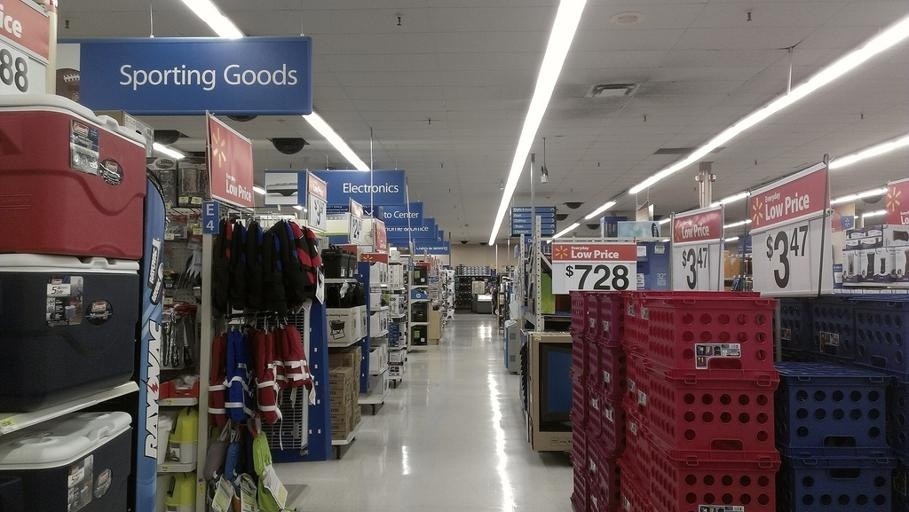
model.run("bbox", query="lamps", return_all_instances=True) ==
[181,1,371,172]
[539,136,550,185]
[548,13,905,244]
[491,3,584,247]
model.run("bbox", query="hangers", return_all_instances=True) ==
[221,215,313,231]
[225,314,295,332]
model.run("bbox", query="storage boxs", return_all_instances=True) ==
[1,408,134,511]
[0,249,143,418]
[526,333,574,452]
[504,321,526,374]
[0,94,150,260]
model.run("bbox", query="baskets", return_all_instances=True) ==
[568,290,909,512]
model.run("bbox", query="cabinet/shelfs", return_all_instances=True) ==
[318,243,411,460]
[412,257,455,352]
[497,264,518,332]
[162,211,211,512]
[518,216,671,332]
